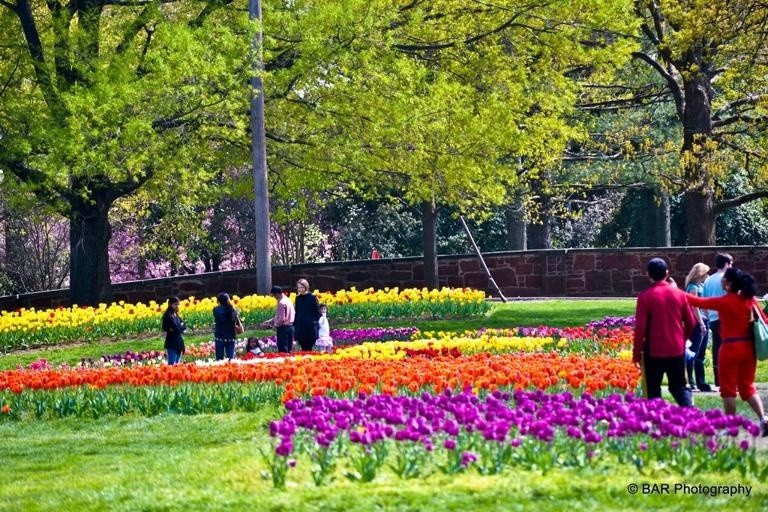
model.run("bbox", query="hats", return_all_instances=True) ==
[648,256,669,281]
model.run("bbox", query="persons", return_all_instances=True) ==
[246,336,263,355]
[270,279,333,353]
[162,295,186,365]
[632,253,768,438]
[213,292,244,360]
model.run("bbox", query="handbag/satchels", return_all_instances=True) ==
[752,305,768,362]
[235,315,245,335]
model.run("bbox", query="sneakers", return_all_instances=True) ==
[760,415,768,438]
